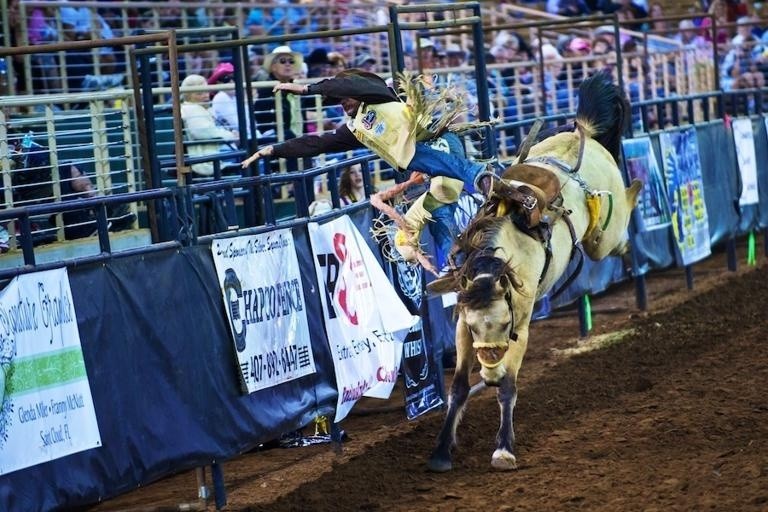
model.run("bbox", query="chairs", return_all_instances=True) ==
[0,1,768,212]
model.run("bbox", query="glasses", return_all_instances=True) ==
[272,56,296,65]
[217,73,236,83]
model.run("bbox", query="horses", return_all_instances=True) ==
[423,63,644,474]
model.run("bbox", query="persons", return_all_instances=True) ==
[3,1,766,276]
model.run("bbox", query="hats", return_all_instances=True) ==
[208,62,234,84]
[353,52,378,69]
[262,46,304,75]
[333,70,388,86]
[413,20,696,61]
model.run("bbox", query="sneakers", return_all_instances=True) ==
[425,269,469,292]
[479,176,494,200]
